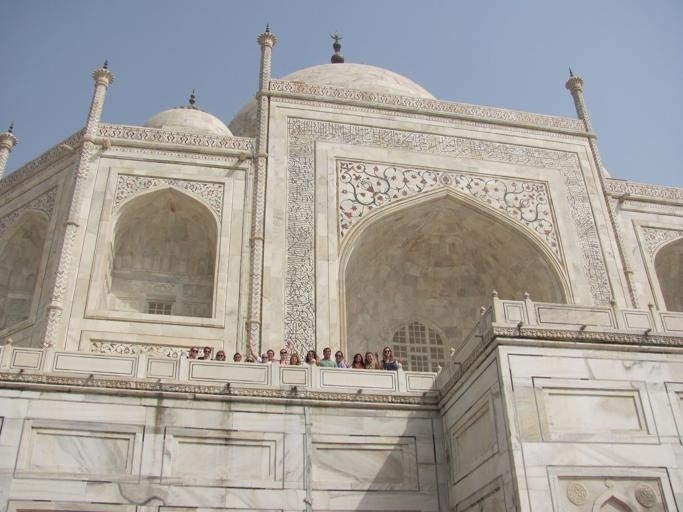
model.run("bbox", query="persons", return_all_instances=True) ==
[186,345,403,371]
